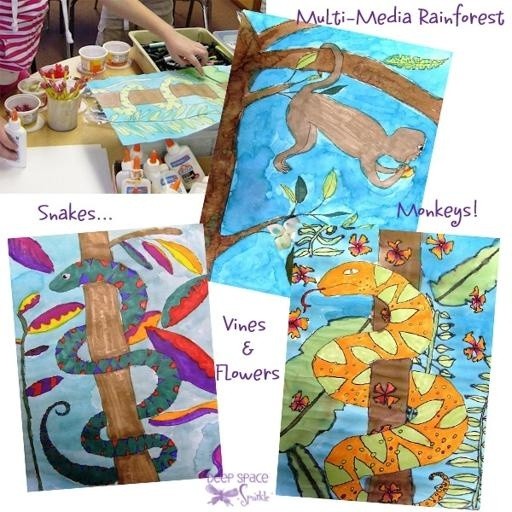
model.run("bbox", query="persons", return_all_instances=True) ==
[0,0,211,162]
[93,0,176,52]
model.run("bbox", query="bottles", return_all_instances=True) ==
[4,109,27,168]
[47,90,79,132]
[116,138,206,194]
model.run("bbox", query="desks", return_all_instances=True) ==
[0,47,212,192]
